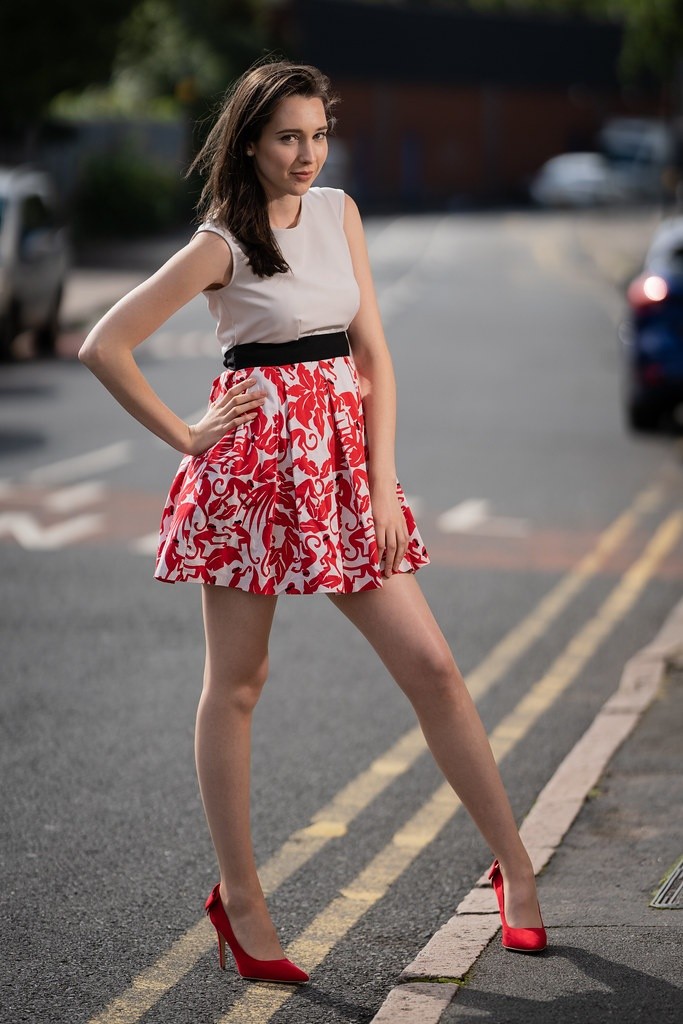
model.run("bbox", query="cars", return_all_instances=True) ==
[622,217,682,432]
[0,168,69,359]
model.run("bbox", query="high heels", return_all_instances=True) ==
[488,858,546,952]
[204,883,308,982]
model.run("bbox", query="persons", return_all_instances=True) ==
[75,61,548,984]
